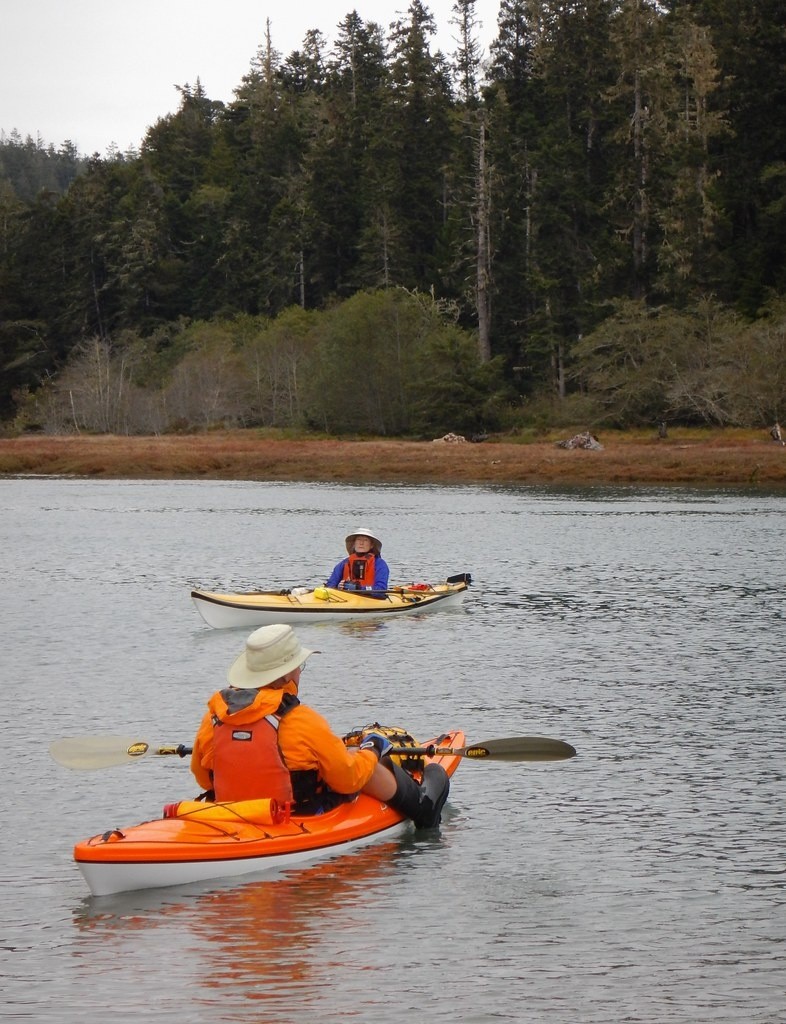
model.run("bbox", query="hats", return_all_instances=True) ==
[227,624,321,689]
[345,528,383,555]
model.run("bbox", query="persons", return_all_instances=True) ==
[190,624,450,830]
[326,528,390,598]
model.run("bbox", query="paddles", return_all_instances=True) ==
[228,589,460,597]
[47,733,580,772]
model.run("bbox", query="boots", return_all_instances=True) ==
[380,755,450,828]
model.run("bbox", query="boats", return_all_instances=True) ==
[75,730,467,896]
[190,573,473,630]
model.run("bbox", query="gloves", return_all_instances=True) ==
[360,733,393,758]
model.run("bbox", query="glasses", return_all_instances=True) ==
[300,662,306,672]
